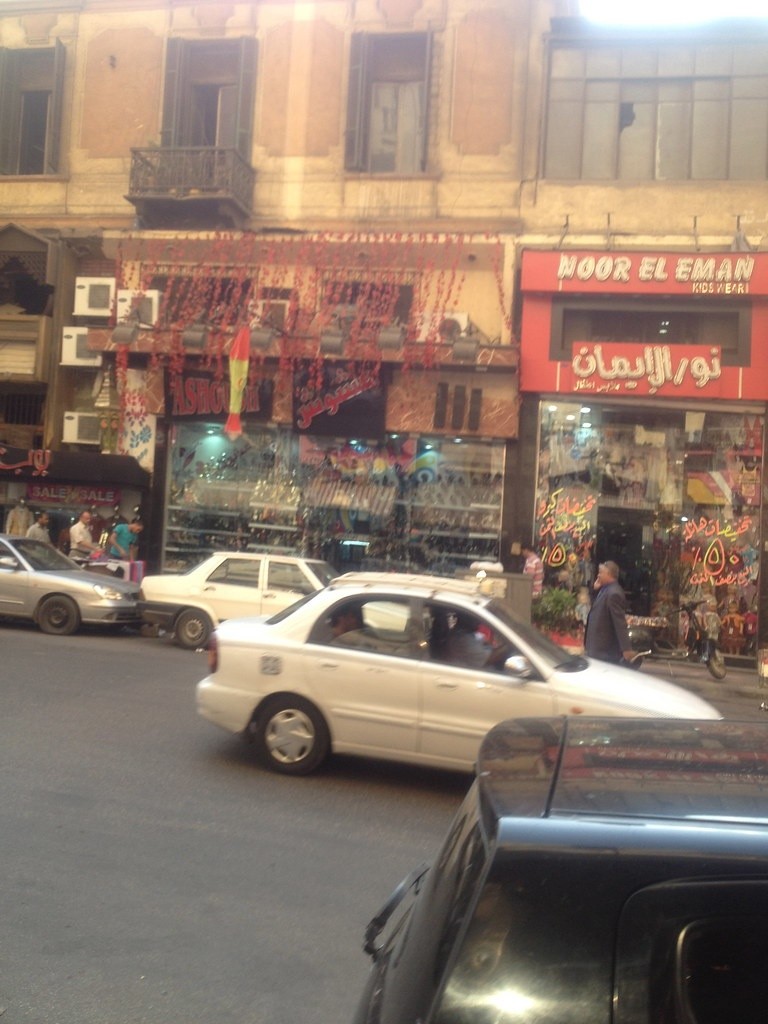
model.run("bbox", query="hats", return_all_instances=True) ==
[605,560,620,577]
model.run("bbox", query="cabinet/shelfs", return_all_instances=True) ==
[164,460,501,577]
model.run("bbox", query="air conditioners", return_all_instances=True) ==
[62,410,101,445]
[117,289,162,331]
[73,277,116,316]
[413,313,469,344]
[59,326,103,367]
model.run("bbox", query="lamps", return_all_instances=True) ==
[247,299,290,333]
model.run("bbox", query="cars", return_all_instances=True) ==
[191,568,723,790]
[0,530,140,635]
[354,710,766,1024]
[134,550,347,647]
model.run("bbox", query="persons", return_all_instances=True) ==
[333,606,358,644]
[584,559,635,665]
[521,542,544,600]
[108,519,144,560]
[5,497,34,537]
[69,512,100,567]
[89,505,106,542]
[26,512,52,545]
[442,614,513,674]
[132,505,141,524]
[107,504,128,535]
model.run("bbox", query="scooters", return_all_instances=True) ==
[615,599,728,679]
